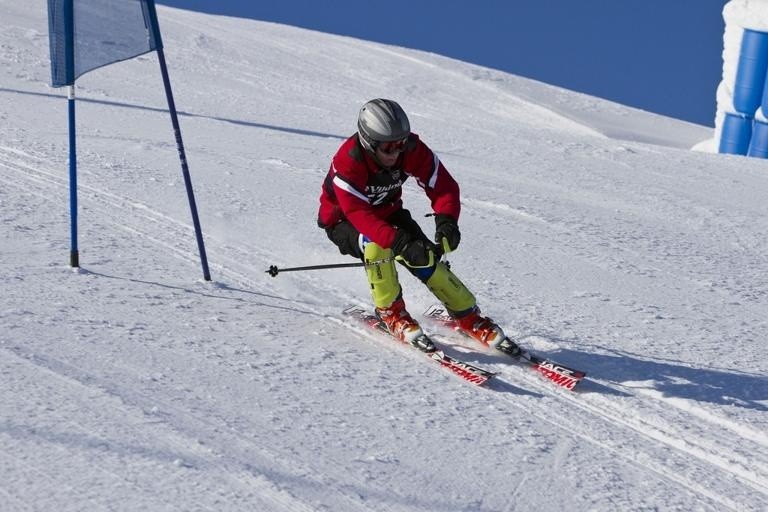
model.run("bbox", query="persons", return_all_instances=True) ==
[315,96,506,353]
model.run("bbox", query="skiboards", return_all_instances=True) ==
[344,303,585,391]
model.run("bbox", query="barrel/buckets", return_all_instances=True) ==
[733,28,768,117]
[760,69,768,118]
[717,112,755,155]
[746,117,768,159]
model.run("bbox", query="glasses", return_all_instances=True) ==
[357,120,410,154]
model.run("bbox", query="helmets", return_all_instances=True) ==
[356,98,409,154]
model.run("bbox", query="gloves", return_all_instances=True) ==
[392,227,437,268]
[434,214,460,254]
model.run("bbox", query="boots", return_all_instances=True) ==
[375,297,422,343]
[454,307,505,347]
